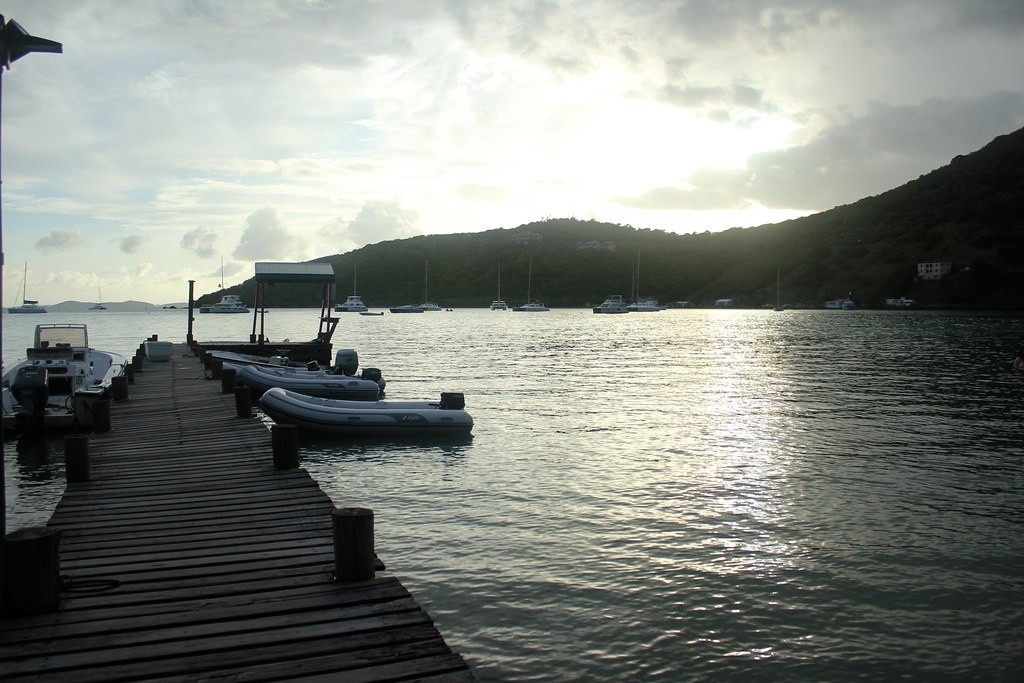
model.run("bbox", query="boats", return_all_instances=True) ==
[259,386,475,434]
[1,322,129,433]
[204,347,359,375]
[824,298,856,310]
[389,304,424,313]
[592,294,630,314]
[235,362,386,401]
[198,294,251,313]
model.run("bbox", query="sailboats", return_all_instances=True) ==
[625,248,660,311]
[490,260,508,310]
[7,261,48,314]
[417,260,443,311]
[87,285,108,310]
[334,262,369,312]
[511,256,550,311]
[773,264,785,312]
